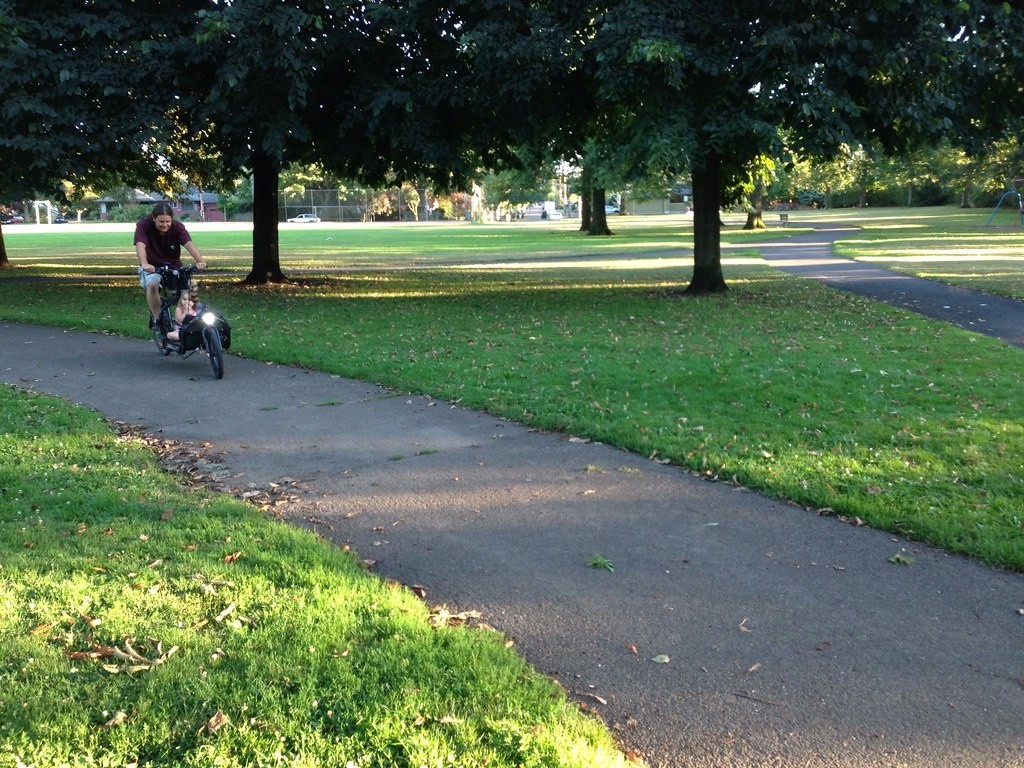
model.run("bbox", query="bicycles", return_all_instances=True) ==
[142,264,225,379]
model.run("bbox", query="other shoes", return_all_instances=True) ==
[154,331,164,348]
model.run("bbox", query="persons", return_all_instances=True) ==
[133,204,207,332]
[0,198,21,221]
[167,291,198,340]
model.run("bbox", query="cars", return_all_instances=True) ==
[548,212,562,220]
[287,213,321,222]
[54,215,69,223]
[605,205,620,214]
[1,215,25,224]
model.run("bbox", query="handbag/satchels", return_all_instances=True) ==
[179,310,230,350]
